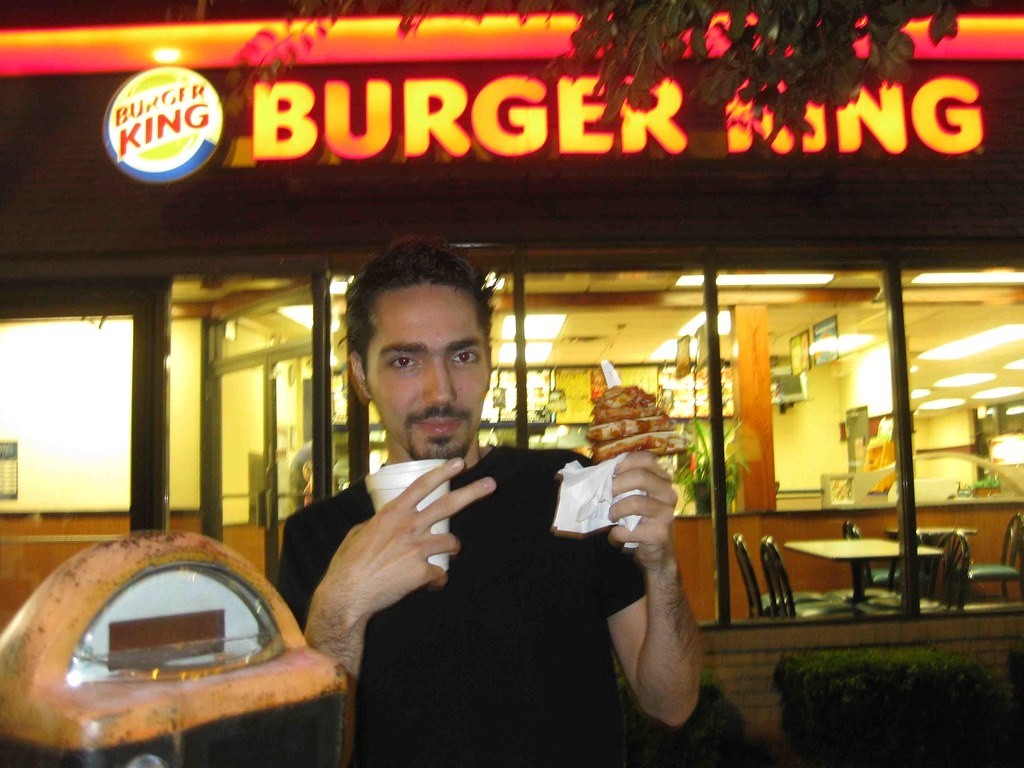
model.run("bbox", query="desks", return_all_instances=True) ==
[783,537,943,617]
[887,528,977,575]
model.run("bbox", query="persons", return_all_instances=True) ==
[277,231,704,768]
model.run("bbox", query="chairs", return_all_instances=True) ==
[732,512,1024,618]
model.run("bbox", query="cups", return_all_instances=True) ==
[363,455,454,574]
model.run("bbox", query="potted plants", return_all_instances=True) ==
[672,417,751,517]
